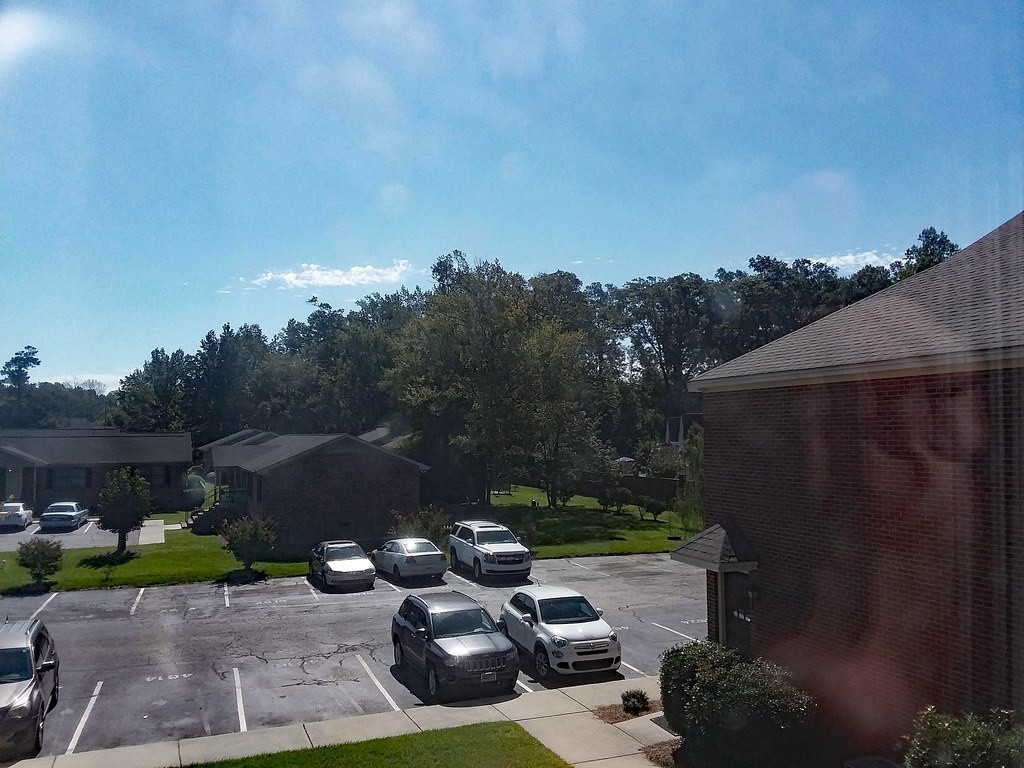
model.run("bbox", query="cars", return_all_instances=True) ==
[499,584,622,681]
[0,502,36,531]
[368,538,448,584]
[39,501,90,531]
[309,539,377,591]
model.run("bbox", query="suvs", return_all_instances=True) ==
[391,590,521,699]
[448,520,533,581]
[0,620,59,754]
